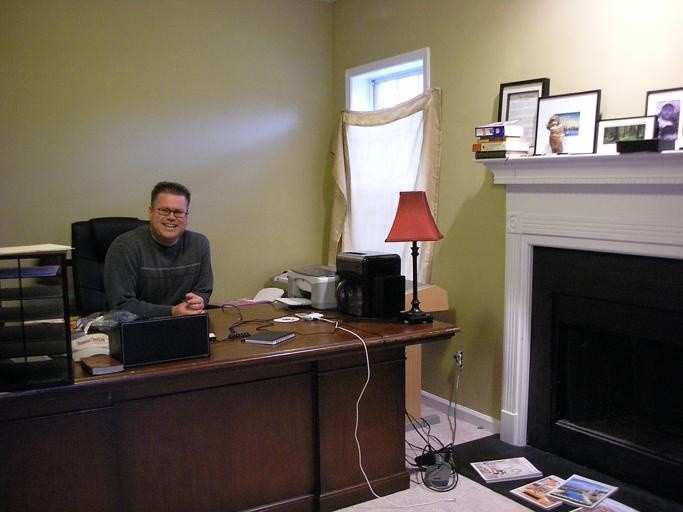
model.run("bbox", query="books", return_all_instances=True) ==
[239,330,296,346]
[81,356,124,376]
[70,333,109,361]
[471,118,530,160]
[468,457,640,511]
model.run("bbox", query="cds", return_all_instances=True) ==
[273,316,300,323]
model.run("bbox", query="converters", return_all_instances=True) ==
[415,453,436,466]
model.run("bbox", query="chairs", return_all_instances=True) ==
[69,215,154,320]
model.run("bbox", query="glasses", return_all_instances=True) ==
[158,208,187,218]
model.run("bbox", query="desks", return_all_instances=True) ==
[2,294,466,511]
[403,279,450,430]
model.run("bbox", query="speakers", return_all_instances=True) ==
[110,313,209,367]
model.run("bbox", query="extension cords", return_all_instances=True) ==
[426,460,451,486]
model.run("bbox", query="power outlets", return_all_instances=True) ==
[450,348,465,373]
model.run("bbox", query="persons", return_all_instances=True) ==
[102,182,214,318]
[545,115,564,154]
[655,103,678,140]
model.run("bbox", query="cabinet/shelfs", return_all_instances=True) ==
[0,244,78,393]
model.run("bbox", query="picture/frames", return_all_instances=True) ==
[495,76,681,160]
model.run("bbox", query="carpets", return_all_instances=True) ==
[415,432,682,512]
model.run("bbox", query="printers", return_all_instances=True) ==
[287,265,340,310]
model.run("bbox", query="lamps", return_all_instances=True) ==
[382,189,445,324]
